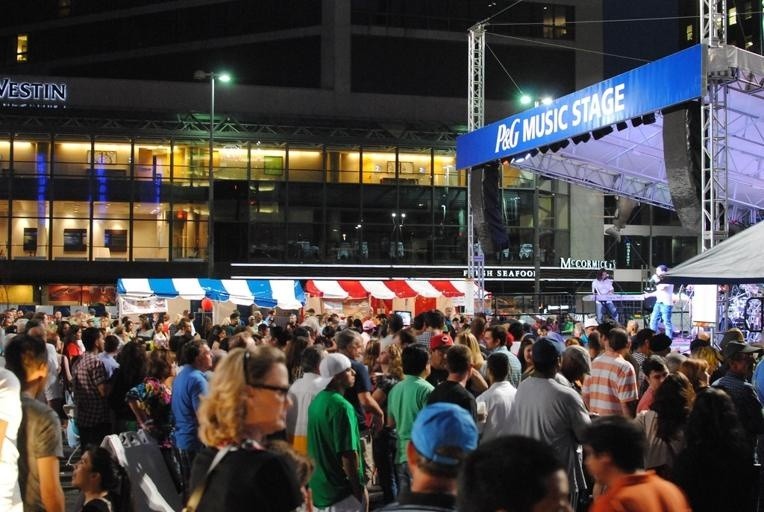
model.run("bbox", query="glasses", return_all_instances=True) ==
[251,383,291,402]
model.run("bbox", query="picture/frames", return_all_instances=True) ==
[263,155,284,177]
[394,310,413,327]
[388,161,400,173]
[400,162,415,173]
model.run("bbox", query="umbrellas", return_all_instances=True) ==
[660,220,760,285]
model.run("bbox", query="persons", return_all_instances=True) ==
[1,306,764,511]
[591,268,619,325]
[640,264,674,340]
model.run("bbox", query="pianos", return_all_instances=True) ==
[582,295,645,301]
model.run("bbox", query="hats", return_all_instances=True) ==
[429,333,455,351]
[314,352,352,390]
[362,320,377,330]
[564,346,592,375]
[198,446,305,510]
[583,317,600,329]
[724,340,762,356]
[410,400,480,468]
[532,337,562,364]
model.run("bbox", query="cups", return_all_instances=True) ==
[477,401,487,423]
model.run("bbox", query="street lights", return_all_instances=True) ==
[391,212,406,263]
[517,92,553,315]
[192,70,231,283]
